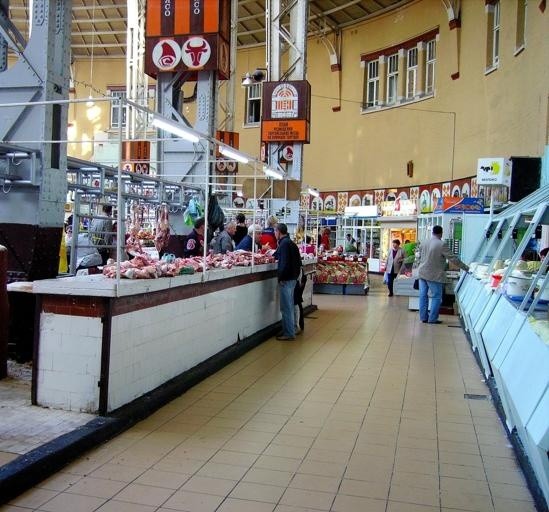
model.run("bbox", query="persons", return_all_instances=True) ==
[88,203,112,257]
[346,233,357,250]
[321,228,332,251]
[539,247,549,266]
[418,225,473,325]
[180,212,278,259]
[65,223,103,274]
[300,235,315,257]
[295,264,307,334]
[402,240,420,272]
[382,238,406,296]
[268,223,302,341]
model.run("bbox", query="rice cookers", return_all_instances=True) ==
[507,274,549,302]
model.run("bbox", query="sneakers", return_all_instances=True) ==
[276,328,301,341]
[422,319,443,324]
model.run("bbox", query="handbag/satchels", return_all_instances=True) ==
[413,279,419,290]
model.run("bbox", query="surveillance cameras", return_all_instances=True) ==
[253,69,264,81]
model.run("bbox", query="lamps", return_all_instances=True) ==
[150,114,284,182]
[240,70,263,86]
[85,0,103,125]
[307,188,320,198]
